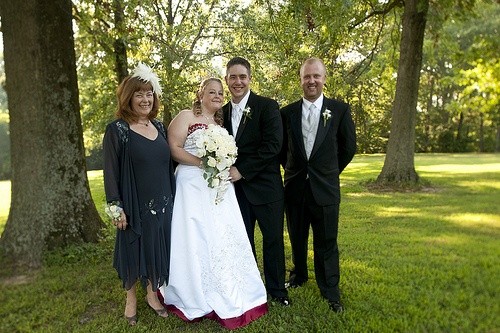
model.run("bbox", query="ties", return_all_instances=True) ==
[307,103,317,126]
[233,105,241,126]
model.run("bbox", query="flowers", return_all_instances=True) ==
[196,122,236,206]
[240,105,253,124]
[322,108,333,126]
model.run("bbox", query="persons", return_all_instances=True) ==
[157,78,269,329]
[102,75,176,326]
[215,56,291,307]
[280,58,357,312]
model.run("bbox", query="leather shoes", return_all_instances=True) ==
[273,298,292,306]
[328,300,343,313]
[284,280,306,289]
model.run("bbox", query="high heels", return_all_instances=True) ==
[144,298,168,318]
[125,300,138,325]
